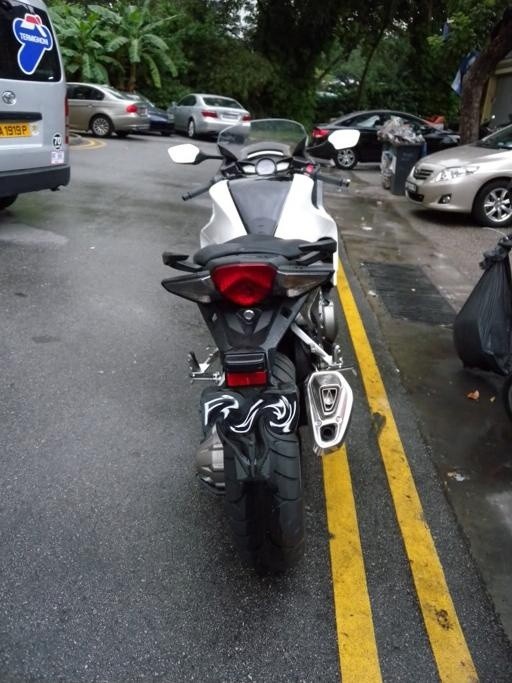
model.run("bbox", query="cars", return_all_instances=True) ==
[403,120,512,227]
[304,110,461,169]
[311,74,358,101]
[61,79,253,140]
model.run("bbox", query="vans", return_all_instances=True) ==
[0,0,77,209]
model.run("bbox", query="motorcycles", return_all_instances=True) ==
[161,112,370,568]
